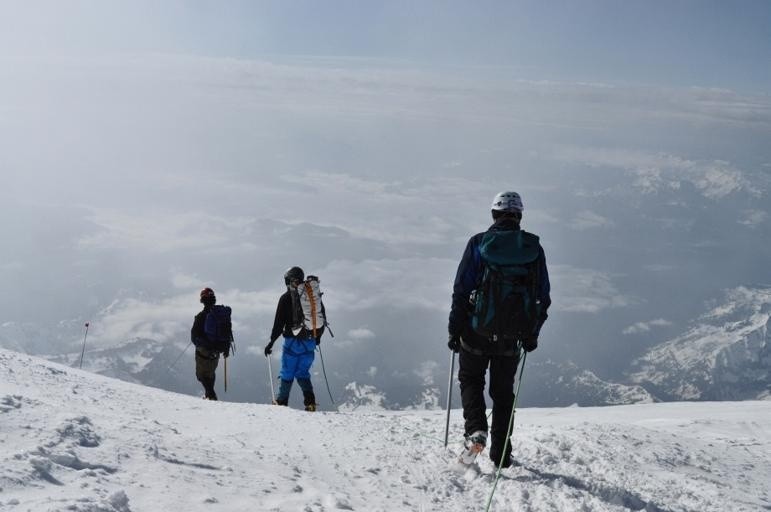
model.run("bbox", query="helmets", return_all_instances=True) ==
[490,190,524,212]
[283,266,304,282]
[200,288,214,298]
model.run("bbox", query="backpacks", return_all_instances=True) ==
[198,305,235,347]
[286,276,326,340]
[467,229,540,350]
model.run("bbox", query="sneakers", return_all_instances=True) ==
[271,400,288,406]
[202,395,218,401]
[305,403,316,411]
[493,456,513,468]
[459,429,487,466]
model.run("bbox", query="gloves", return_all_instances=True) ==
[449,334,462,354]
[223,346,230,359]
[264,341,273,358]
[313,336,321,346]
[520,336,538,352]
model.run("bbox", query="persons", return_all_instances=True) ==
[447,189,553,470]
[190,287,233,400]
[264,265,327,412]
